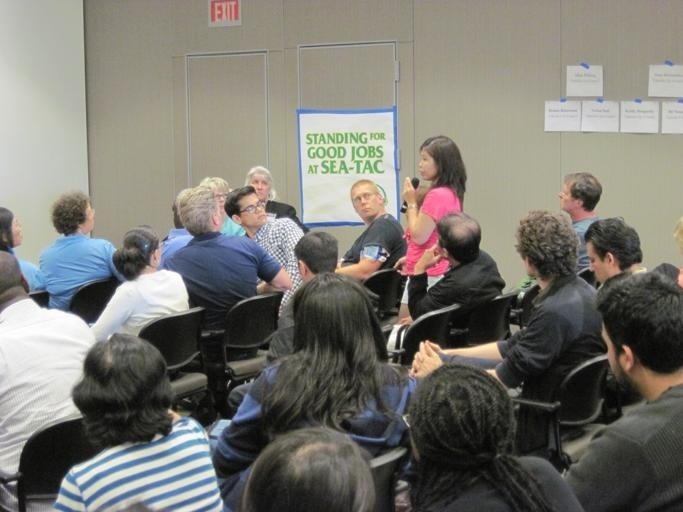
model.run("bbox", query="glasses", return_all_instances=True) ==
[237,200,263,214]
[213,193,227,198]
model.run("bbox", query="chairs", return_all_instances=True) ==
[462,292,520,347]
[28,290,50,309]
[512,281,541,325]
[577,267,599,290]
[69,276,123,328]
[0,414,105,512]
[202,291,284,419]
[363,268,408,321]
[369,445,409,512]
[510,353,609,474]
[387,303,462,365]
[138,306,218,428]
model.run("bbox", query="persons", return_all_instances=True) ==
[157,201,193,270]
[558,172,601,273]
[408,362,585,512]
[31,191,126,313]
[240,427,376,512]
[200,177,245,236]
[244,166,309,235]
[332,179,408,283]
[90,224,190,341]
[410,208,608,450]
[224,186,305,316]
[0,206,38,291]
[228,231,348,415]
[392,135,466,323]
[51,333,224,512]
[162,186,292,364]
[386,211,507,364]
[585,216,647,289]
[561,271,683,512]
[0,251,96,482]
[203,271,417,509]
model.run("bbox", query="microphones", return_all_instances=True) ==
[399,177,420,213]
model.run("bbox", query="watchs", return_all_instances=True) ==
[407,203,417,208]
